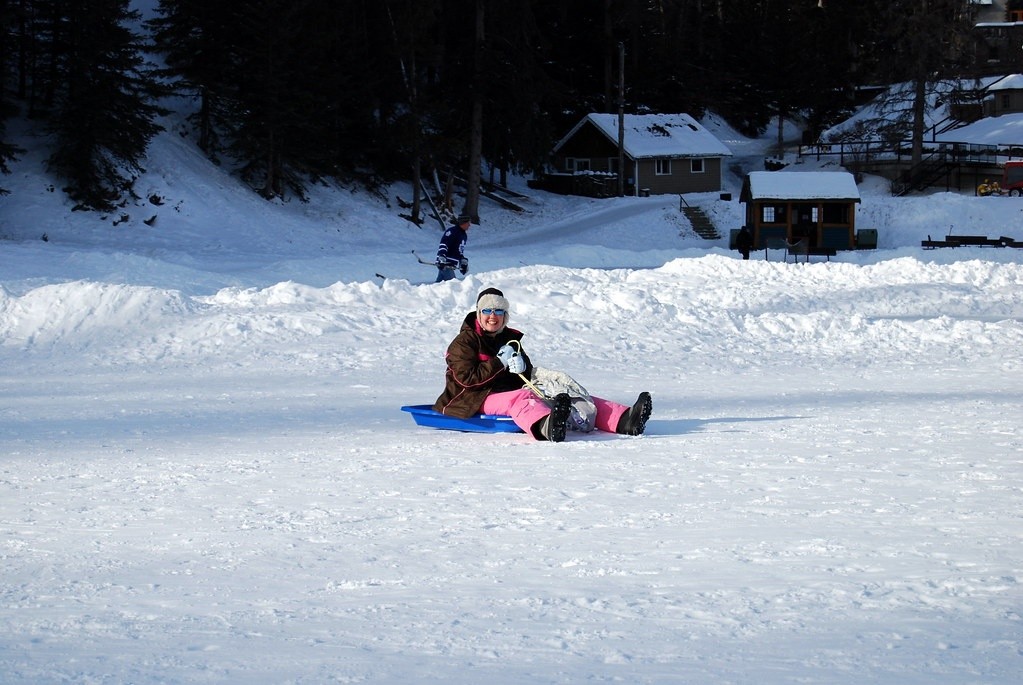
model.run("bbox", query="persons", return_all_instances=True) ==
[435,213,472,283]
[432,288,652,442]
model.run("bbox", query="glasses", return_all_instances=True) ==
[482,309,504,315]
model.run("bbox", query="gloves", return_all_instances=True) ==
[507,351,526,374]
[459,258,468,275]
[437,256,446,270]
[496,345,514,370]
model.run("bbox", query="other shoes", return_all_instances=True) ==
[539,393,571,442]
[617,392,653,435]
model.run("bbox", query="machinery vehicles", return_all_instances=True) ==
[976,179,1010,197]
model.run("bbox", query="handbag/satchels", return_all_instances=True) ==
[522,367,597,433]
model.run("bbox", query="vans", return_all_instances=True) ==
[1002,162,1023,197]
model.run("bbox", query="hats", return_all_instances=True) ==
[476,288,509,333]
[458,213,472,224]
